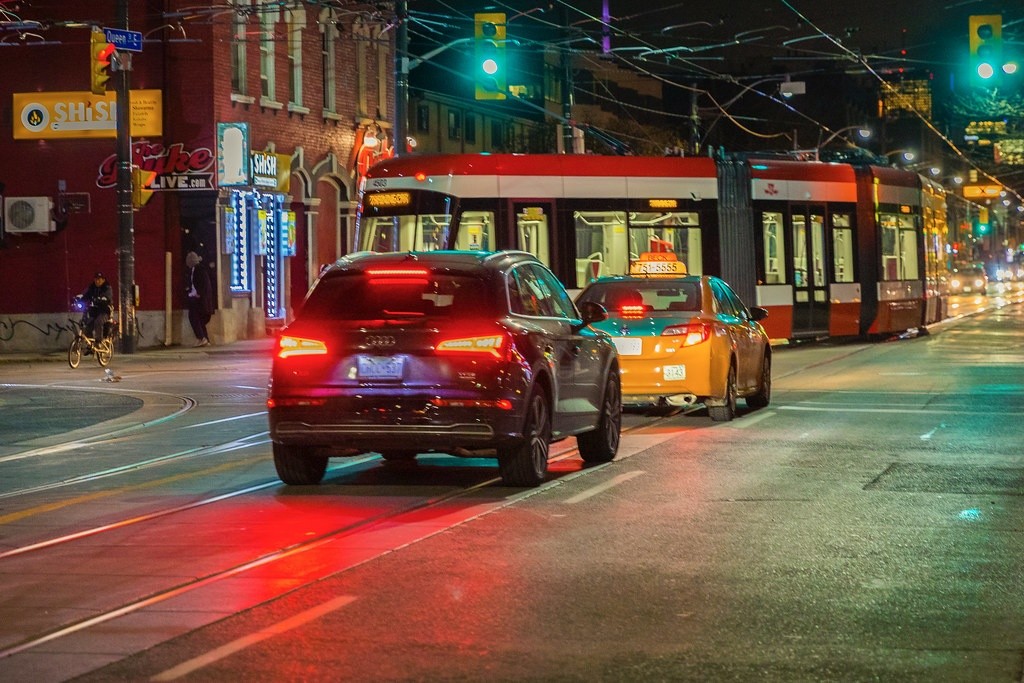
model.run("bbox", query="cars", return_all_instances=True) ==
[573,252,772,422]
[985,250,1024,282]
[949,264,988,297]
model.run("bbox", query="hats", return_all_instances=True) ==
[94,272,105,279]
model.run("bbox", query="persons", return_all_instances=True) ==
[184,251,216,347]
[74,272,113,356]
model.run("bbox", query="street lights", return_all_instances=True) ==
[688,76,791,153]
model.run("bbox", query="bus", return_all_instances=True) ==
[349,135,950,348]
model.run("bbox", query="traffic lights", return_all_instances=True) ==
[475,12,506,99]
[90,30,116,97]
[968,14,1002,88]
[978,222,987,234]
[1001,54,1021,81]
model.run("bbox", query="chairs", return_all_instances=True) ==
[669,302,686,311]
[450,280,488,319]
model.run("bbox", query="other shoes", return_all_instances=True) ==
[205,343,211,346]
[195,337,208,347]
[95,341,101,348]
[84,349,91,356]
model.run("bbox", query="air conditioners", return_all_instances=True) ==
[5,196,56,235]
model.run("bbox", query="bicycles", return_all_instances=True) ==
[68,297,119,370]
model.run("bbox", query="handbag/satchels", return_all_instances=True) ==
[103,321,119,338]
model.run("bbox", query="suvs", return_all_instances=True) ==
[265,250,623,489]
